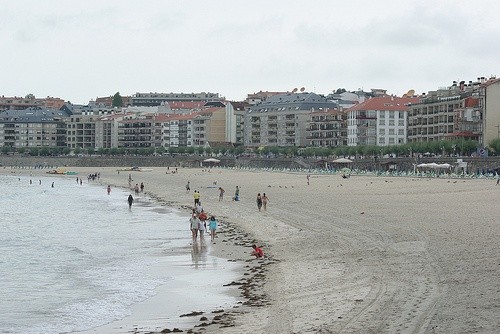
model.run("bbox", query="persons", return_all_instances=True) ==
[342,171,347,178]
[496,169,500,184]
[30,163,310,258]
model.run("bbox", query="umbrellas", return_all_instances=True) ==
[418,162,454,168]
[333,158,353,164]
[204,158,220,163]
